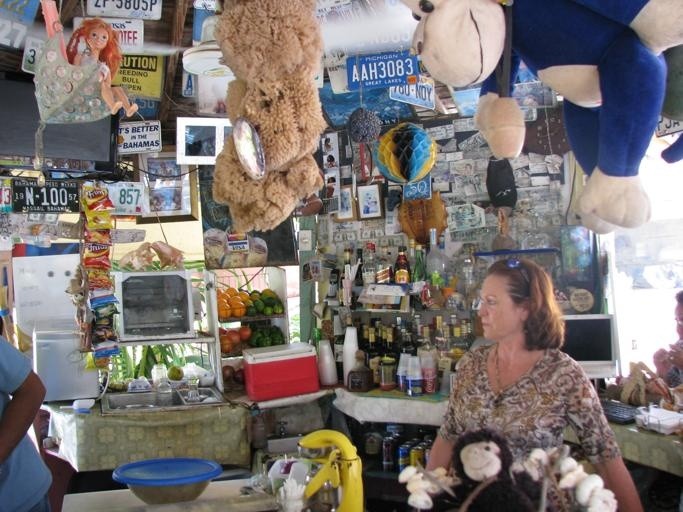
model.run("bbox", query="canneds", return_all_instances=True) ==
[383,434,433,470]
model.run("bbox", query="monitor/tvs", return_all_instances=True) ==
[557,314,617,367]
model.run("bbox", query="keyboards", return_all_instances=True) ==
[597,398,636,424]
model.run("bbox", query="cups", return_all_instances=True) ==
[341,324,361,388]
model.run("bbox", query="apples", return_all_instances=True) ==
[167,366,184,380]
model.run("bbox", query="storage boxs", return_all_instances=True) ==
[33,318,100,402]
[241,342,320,401]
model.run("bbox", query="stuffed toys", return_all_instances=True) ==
[397,0,683,234]
[209,0,325,237]
[396,428,617,512]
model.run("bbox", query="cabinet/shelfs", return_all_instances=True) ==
[205,264,291,393]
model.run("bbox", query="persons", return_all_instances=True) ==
[425,259,643,512]
[31,18,140,126]
[1,336,56,511]
[652,290,683,408]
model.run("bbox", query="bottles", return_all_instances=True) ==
[319,340,337,386]
[394,246,411,284]
[376,245,390,284]
[426,228,442,276]
[413,245,426,282]
[362,243,376,284]
[151,364,167,386]
[333,310,475,396]
[339,248,352,307]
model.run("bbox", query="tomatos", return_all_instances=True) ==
[218,325,252,353]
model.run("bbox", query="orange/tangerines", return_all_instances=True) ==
[248,325,284,346]
[247,290,282,315]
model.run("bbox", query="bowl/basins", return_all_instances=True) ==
[169,378,187,386]
[113,458,223,504]
[127,380,152,393]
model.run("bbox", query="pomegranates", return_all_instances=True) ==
[222,365,244,383]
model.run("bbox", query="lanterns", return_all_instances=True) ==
[371,119,438,186]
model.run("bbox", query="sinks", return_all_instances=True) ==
[100,390,184,413]
[176,388,221,405]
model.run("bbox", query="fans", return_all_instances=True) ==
[115,2,416,105]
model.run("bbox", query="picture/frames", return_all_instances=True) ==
[175,116,233,166]
[333,183,358,222]
[133,146,199,224]
[355,181,386,222]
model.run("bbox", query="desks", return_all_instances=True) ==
[334,385,452,429]
[40,398,252,474]
[58,480,294,512]
[562,404,683,480]
